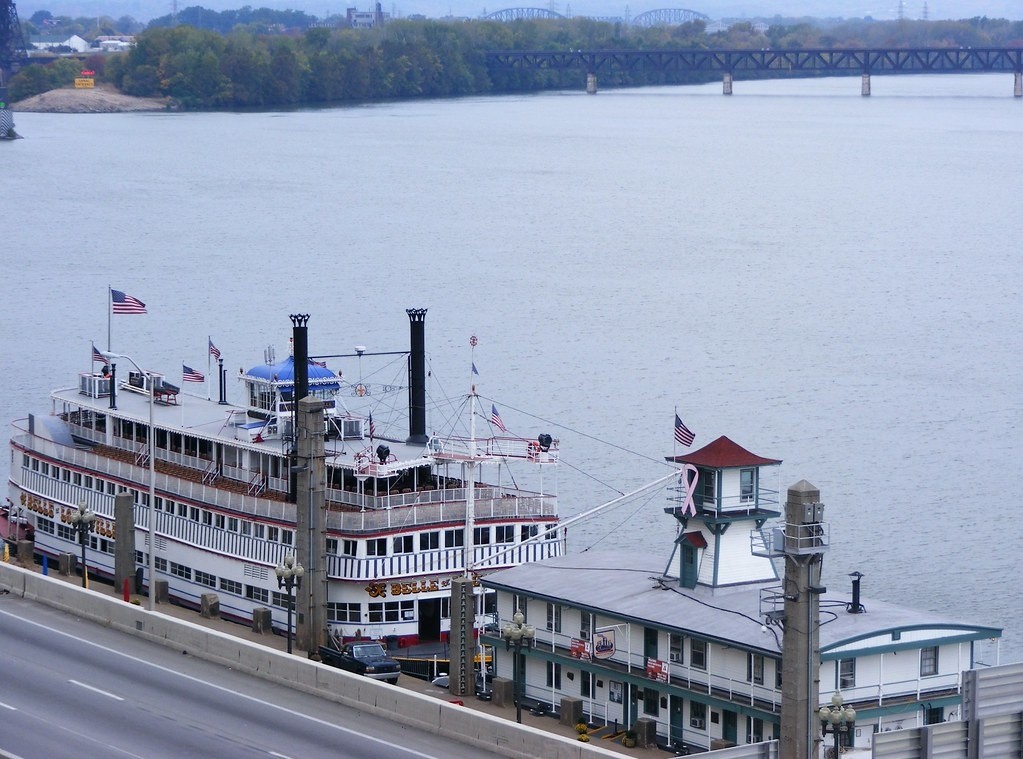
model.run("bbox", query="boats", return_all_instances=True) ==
[475,434,1004,759]
[8,283,569,651]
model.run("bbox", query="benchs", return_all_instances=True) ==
[237,421,277,442]
[153,381,180,406]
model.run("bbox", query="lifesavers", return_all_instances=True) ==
[528,441,540,456]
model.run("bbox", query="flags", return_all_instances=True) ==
[93,345,109,364]
[492,402,506,432]
[111,289,148,315]
[183,365,205,383]
[370,412,375,441]
[210,341,221,362]
[674,413,695,447]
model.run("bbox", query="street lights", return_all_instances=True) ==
[275,552,305,655]
[503,608,534,724]
[70,502,95,590]
[100,351,156,611]
[818,689,856,759]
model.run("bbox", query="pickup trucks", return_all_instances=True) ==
[317,641,401,685]
